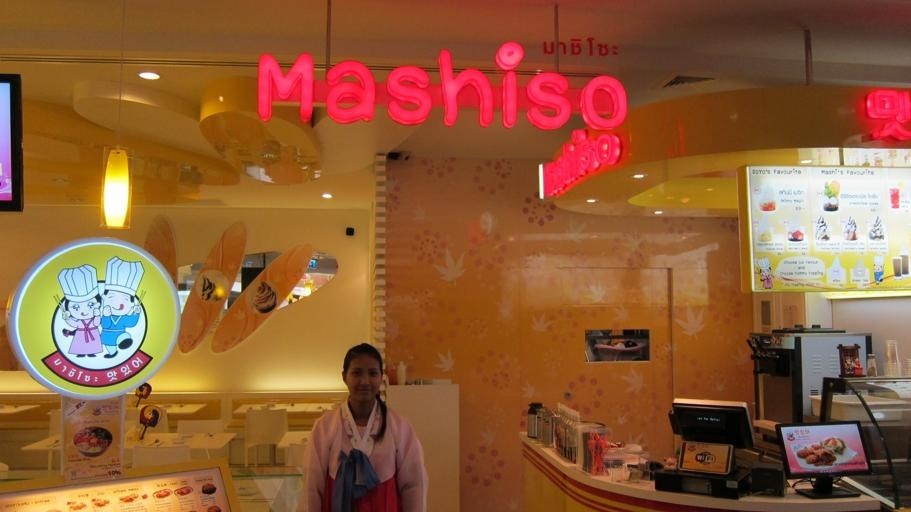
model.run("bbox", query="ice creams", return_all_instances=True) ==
[842,216,858,240]
[814,215,829,241]
[868,215,885,239]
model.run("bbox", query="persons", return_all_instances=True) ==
[305,340,429,512]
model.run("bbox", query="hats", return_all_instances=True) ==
[57,264,100,303]
[104,256,145,296]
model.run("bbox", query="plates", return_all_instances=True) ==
[790,436,857,469]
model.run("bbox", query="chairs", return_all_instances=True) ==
[25,405,312,468]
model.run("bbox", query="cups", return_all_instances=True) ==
[874,255,885,286]
[883,339,911,379]
[889,188,900,209]
[898,254,910,277]
[892,256,903,280]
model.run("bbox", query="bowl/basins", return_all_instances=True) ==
[593,341,647,361]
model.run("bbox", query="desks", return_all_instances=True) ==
[1,404,38,473]
[233,403,335,464]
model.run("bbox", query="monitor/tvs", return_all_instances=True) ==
[668,397,757,451]
[775,420,874,499]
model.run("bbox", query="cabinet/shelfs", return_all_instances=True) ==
[818,377,910,512]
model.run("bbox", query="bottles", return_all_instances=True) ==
[525,402,544,439]
[395,360,408,385]
[866,353,878,377]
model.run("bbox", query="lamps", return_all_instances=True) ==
[101,16,133,230]
